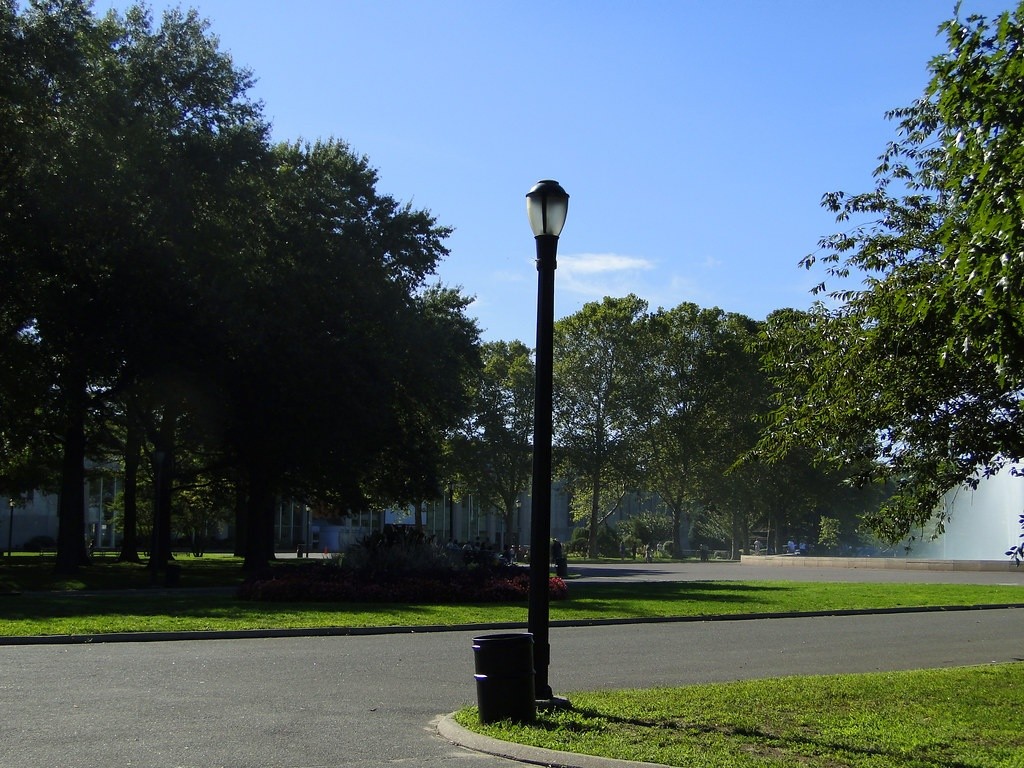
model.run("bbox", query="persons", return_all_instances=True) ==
[499,544,515,566]
[656,541,662,552]
[620,542,626,560]
[788,537,795,553]
[754,539,761,556]
[632,542,638,560]
[297,545,303,558]
[88,540,96,557]
[799,540,808,555]
[431,535,489,556]
[645,541,652,562]
[699,542,708,561]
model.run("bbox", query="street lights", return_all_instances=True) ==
[447,478,458,541]
[525,179,570,699]
[516,499,522,547]
[306,504,312,558]
[8,498,16,556]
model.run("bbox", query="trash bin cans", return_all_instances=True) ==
[556,564,568,577]
[471,631,537,725]
